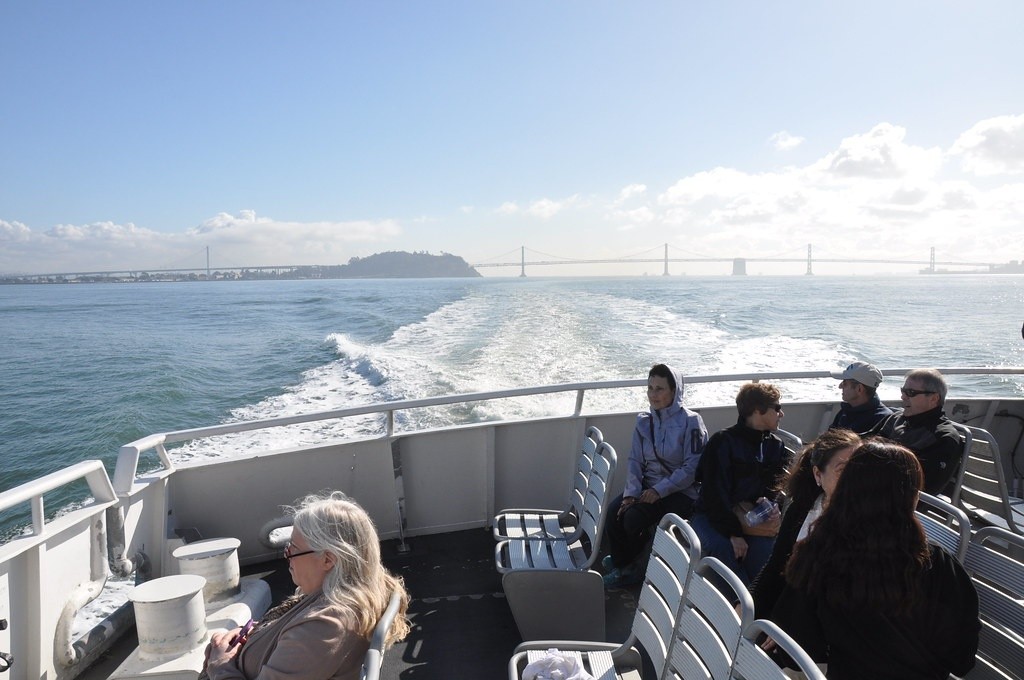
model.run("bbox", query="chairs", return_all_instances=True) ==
[355,422,1024,680]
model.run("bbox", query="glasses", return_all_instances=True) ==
[901,387,941,400]
[282,538,315,559]
[768,401,782,411]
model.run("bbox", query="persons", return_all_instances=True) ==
[207,498,410,680]
[605,359,981,680]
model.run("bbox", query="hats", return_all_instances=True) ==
[832,362,883,389]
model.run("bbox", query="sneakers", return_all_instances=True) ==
[602,555,645,588]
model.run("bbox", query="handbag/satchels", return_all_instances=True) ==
[734,499,781,536]
